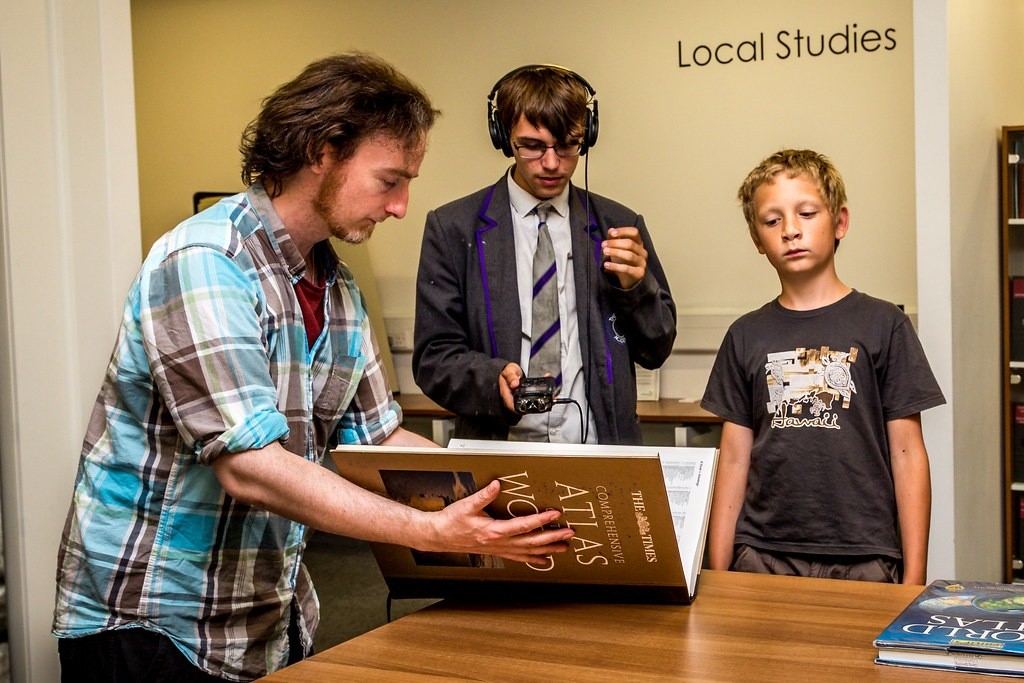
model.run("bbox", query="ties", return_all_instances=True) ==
[528,202,563,397]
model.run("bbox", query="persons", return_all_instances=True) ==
[411,65,678,446]
[705,148,933,585]
[52,47,576,683]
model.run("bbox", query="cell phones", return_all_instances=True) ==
[513,376,556,415]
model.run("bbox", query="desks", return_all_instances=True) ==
[251,569,1024,683]
[387,393,729,448]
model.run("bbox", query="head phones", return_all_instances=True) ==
[487,64,599,158]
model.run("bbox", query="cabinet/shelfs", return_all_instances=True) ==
[999,125,1024,585]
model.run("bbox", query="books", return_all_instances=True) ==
[327,436,721,602]
[873,580,1024,678]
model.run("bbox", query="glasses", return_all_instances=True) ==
[510,130,583,160]
